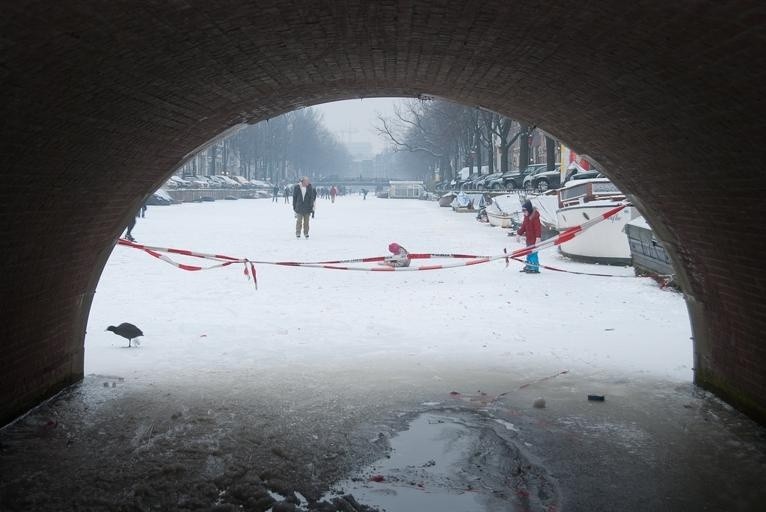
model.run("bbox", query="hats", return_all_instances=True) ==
[521,200,532,215]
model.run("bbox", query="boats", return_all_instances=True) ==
[438,178,640,266]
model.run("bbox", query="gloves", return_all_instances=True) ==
[516,234,521,243]
[535,237,541,244]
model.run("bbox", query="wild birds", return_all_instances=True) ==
[104,323,144,348]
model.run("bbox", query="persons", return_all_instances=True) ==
[136,203,145,218]
[272,180,372,203]
[292,176,317,238]
[515,200,542,273]
[124,220,136,242]
[377,242,411,267]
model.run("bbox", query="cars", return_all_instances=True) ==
[166,175,274,189]
[436,163,606,193]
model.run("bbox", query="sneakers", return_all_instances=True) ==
[523,266,538,271]
[296,235,301,238]
[305,233,309,237]
